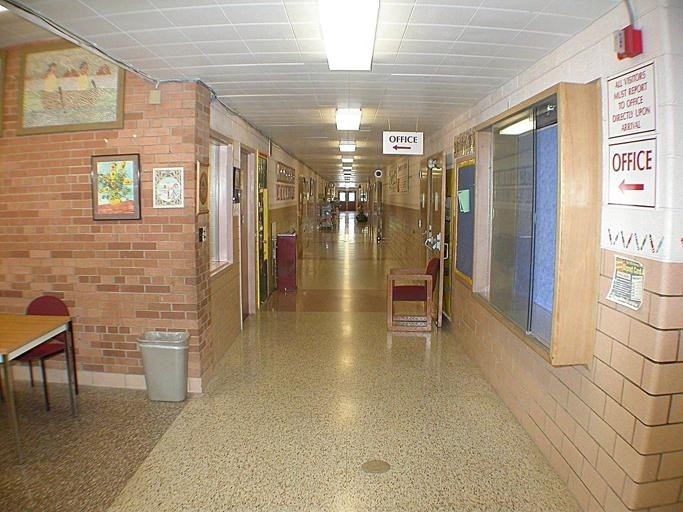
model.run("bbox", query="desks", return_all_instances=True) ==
[0,312,77,466]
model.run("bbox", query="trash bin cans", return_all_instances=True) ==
[136,331,191,402]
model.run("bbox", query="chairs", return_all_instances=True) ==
[384,258,440,332]
[11,295,79,411]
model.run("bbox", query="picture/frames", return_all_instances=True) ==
[15,41,125,136]
[90,152,141,221]
[151,166,185,209]
[196,160,210,216]
